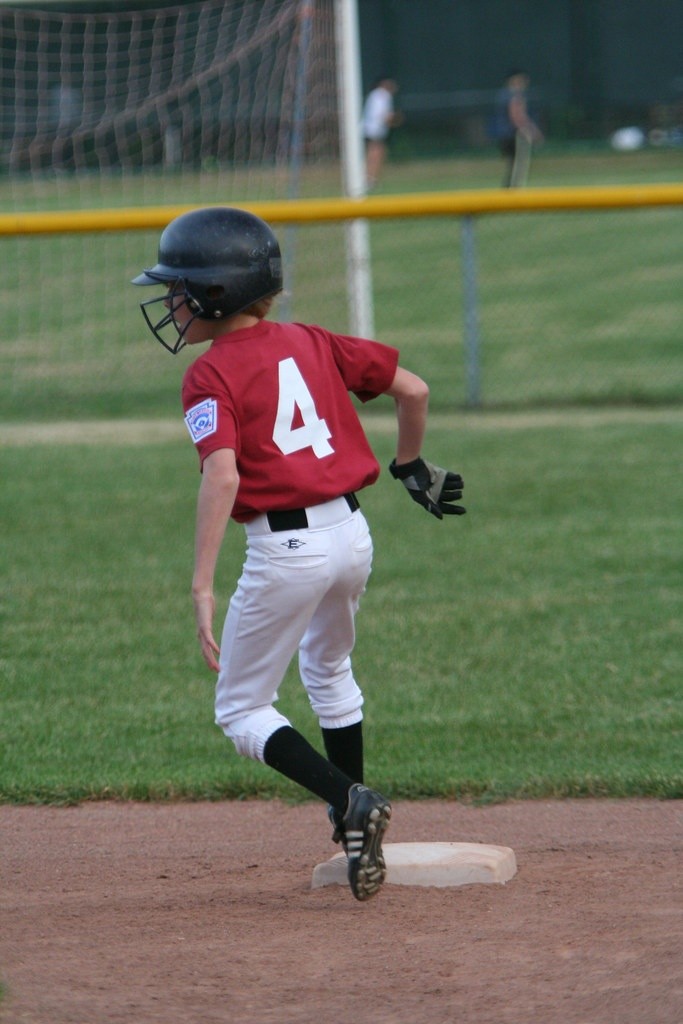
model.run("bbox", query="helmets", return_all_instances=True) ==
[129,208,284,321]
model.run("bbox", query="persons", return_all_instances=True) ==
[132,206,465,902]
[495,71,543,187]
[360,75,404,182]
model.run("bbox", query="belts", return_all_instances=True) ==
[266,491,360,533]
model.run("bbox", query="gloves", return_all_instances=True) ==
[389,456,467,520]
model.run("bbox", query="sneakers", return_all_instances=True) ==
[327,782,392,901]
